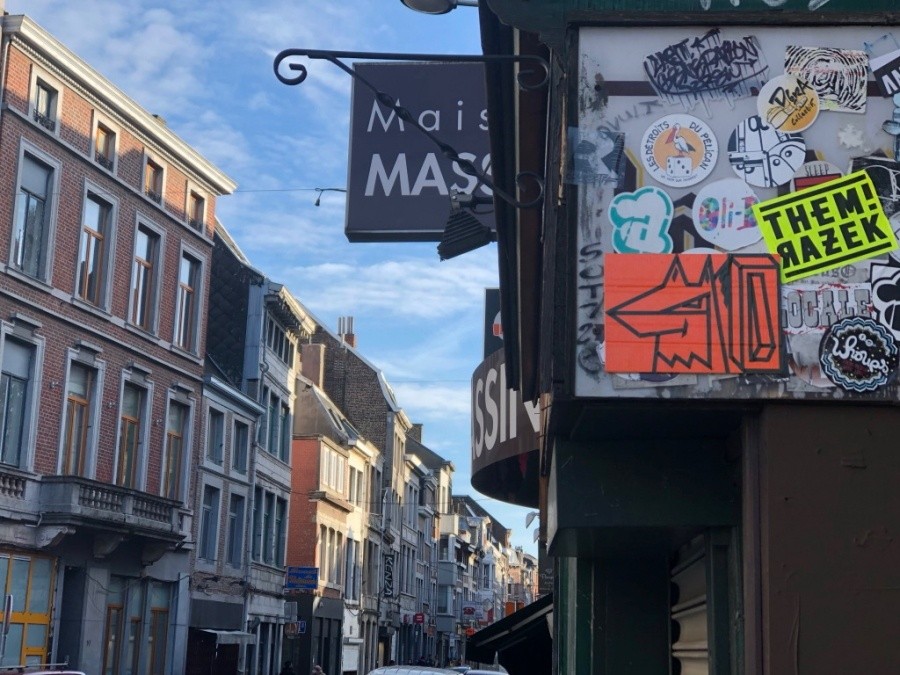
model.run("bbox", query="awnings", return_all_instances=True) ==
[462,593,554,667]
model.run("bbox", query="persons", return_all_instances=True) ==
[279,659,296,675]
[388,655,463,669]
[310,664,327,675]
